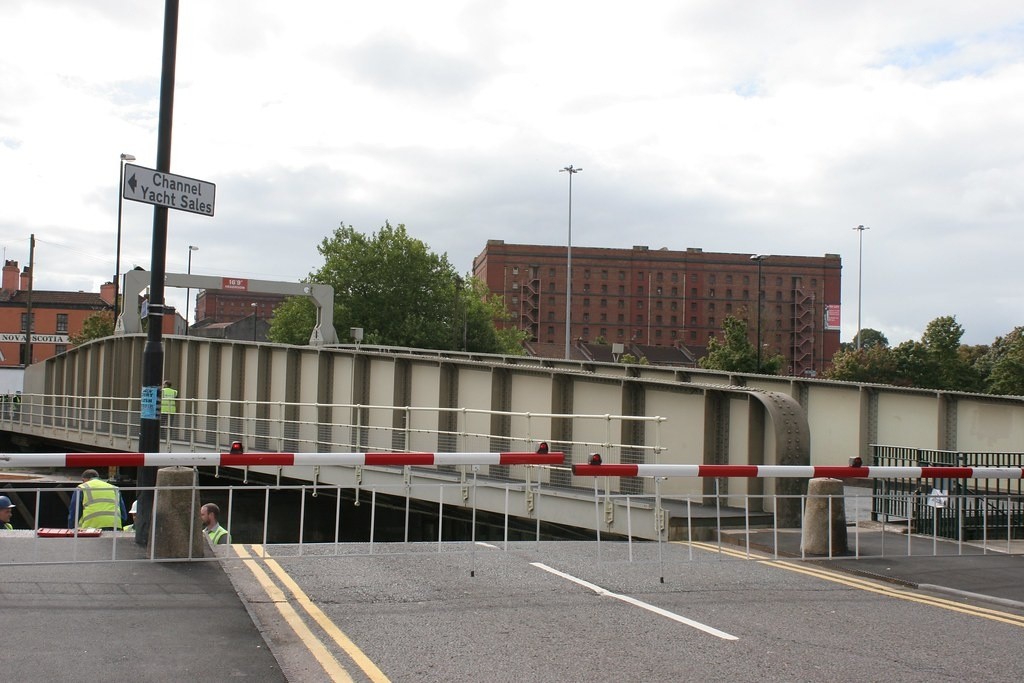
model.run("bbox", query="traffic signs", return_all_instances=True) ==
[124,163,217,217]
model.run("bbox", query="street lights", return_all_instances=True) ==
[749,254,771,369]
[852,225,870,351]
[559,165,583,359]
[185,245,199,333]
[251,302,258,341]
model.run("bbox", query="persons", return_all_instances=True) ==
[200,502,232,544]
[0,495,14,529]
[123,499,138,533]
[13,390,21,420]
[161,380,178,439]
[68,469,127,531]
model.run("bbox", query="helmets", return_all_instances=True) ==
[0,496,16,508]
[15,391,21,395]
[128,500,138,513]
